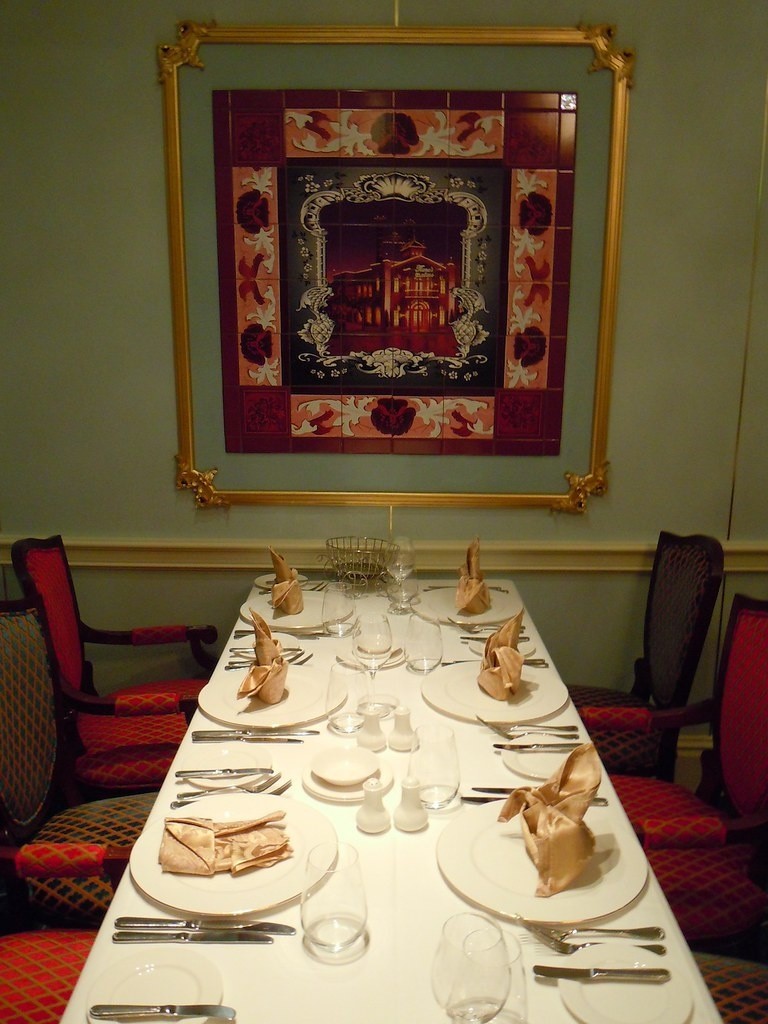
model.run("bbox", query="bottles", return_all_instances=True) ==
[357,707,429,834]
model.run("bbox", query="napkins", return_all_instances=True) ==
[497,743,604,899]
[476,606,526,701]
[265,544,304,616]
[455,535,493,615]
[156,810,293,878]
[236,605,290,706]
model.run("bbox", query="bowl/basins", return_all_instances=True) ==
[313,744,381,786]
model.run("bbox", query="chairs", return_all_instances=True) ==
[693,951,768,1024]
[0,843,133,1024]
[10,532,222,793]
[0,595,160,919]
[564,530,725,785]
[577,592,768,949]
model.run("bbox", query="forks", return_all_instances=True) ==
[171,773,292,810]
[448,618,526,633]
[225,651,313,671]
[476,716,579,739]
[520,919,666,955]
[259,582,328,595]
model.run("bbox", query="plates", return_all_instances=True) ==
[86,949,223,1024]
[411,587,537,655]
[422,661,568,723]
[130,794,339,915]
[255,573,308,588]
[303,639,407,801]
[437,799,648,923]
[240,591,353,628]
[198,665,347,728]
[558,944,694,1024]
[502,735,572,779]
[235,633,299,657]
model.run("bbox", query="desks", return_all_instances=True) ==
[55,578,726,1024]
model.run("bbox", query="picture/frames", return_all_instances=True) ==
[151,18,638,519]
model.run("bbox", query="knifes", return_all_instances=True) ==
[90,917,296,1019]
[266,580,308,583]
[229,630,331,652]
[533,965,671,981]
[442,636,549,668]
[461,743,608,807]
[175,729,320,777]
[423,585,508,592]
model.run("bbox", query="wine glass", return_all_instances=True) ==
[354,611,393,718]
[385,536,414,615]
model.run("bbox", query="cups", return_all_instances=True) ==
[328,664,374,733]
[432,913,528,1024]
[409,724,459,807]
[301,842,368,949]
[323,565,442,670]
